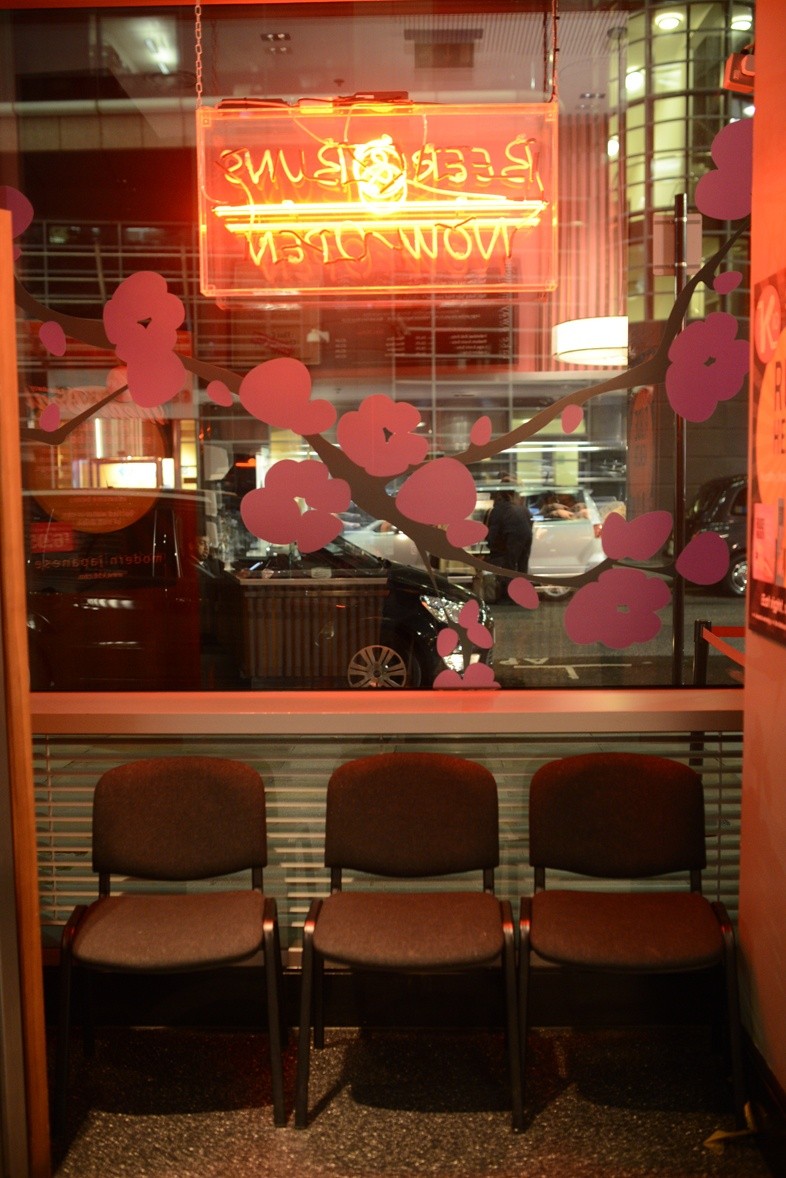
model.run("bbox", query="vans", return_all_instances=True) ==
[343,486,609,603]
[23,488,496,690]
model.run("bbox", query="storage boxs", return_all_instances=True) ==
[226,566,389,681]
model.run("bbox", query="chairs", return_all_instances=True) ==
[517,751,740,1115]
[56,755,289,1129]
[294,752,520,1130]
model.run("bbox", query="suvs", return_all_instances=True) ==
[686,475,748,596]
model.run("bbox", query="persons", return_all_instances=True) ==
[191,530,249,579]
[483,474,533,600]
